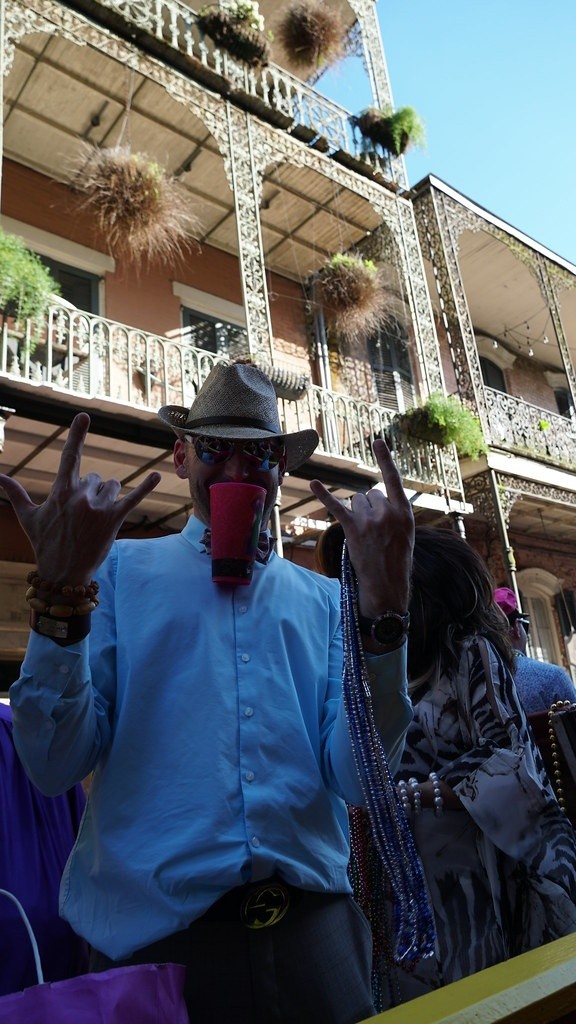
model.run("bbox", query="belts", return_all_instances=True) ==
[193,884,345,930]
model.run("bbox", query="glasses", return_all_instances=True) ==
[182,434,285,470]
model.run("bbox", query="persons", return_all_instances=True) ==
[317,521,575,989]
[0,360,576,1024]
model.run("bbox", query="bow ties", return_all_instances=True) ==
[200,528,277,565]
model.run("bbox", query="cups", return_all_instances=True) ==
[210,482,267,585]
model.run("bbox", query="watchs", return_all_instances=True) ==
[356,612,411,646]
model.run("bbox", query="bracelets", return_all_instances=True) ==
[26,570,99,614]
[394,772,443,816]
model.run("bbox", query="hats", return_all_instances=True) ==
[494,587,530,625]
[157,359,318,472]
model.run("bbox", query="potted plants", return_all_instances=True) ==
[274,0,342,63]
[303,251,389,336]
[0,226,63,340]
[91,146,192,264]
[361,108,420,156]
[400,390,490,459]
[199,0,271,64]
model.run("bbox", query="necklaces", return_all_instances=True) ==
[336,537,436,1014]
[548,700,570,812]
